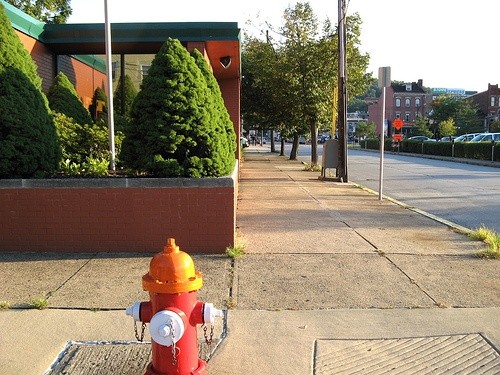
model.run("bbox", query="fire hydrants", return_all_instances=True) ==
[125,237,222,375]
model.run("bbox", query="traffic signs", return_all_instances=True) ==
[392,118,404,130]
[394,134,403,142]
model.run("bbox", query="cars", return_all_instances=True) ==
[438,137,455,143]
[453,134,477,141]
[408,136,436,142]
[470,132,500,143]
[297,138,307,144]
